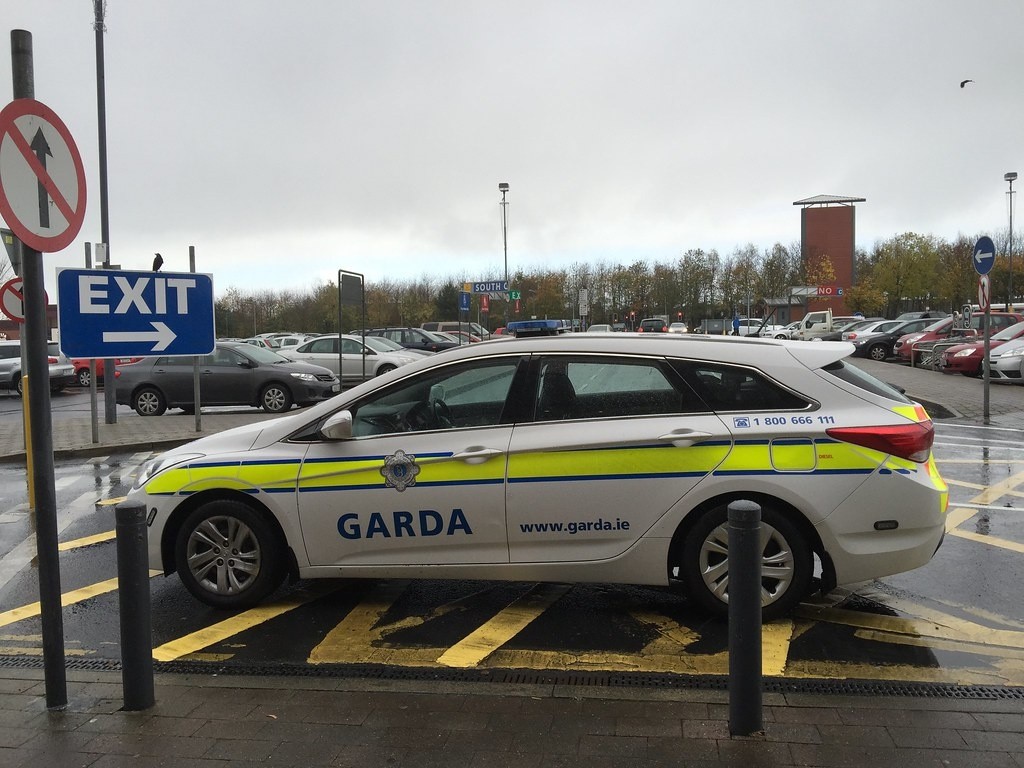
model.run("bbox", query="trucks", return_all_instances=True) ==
[798,307,866,342]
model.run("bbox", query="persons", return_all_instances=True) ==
[733,316,741,336]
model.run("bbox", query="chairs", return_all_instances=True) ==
[538,366,582,420]
[228,352,238,364]
[344,342,354,353]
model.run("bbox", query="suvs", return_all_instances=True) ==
[1,339,77,396]
[893,311,1024,362]
[349,327,461,354]
[638,319,667,333]
[420,320,515,340]
[729,318,784,336]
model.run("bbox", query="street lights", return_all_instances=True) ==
[499,183,509,282]
[1004,171,1017,309]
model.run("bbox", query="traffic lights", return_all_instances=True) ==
[613,313,619,322]
[630,312,636,322]
[678,312,682,321]
[624,316,629,321]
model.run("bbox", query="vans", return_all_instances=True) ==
[972,302,1024,316]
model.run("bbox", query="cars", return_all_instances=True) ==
[612,322,628,332]
[115,343,342,415]
[811,317,887,342]
[216,333,338,355]
[276,335,436,382]
[493,327,516,336]
[668,322,689,334]
[586,324,614,332]
[980,336,1024,386]
[761,321,802,340]
[119,317,947,619]
[941,320,1024,377]
[850,318,943,361]
[841,320,906,342]
[446,331,481,342]
[70,357,141,387]
[894,311,947,333]
[430,331,463,344]
[694,326,703,333]
